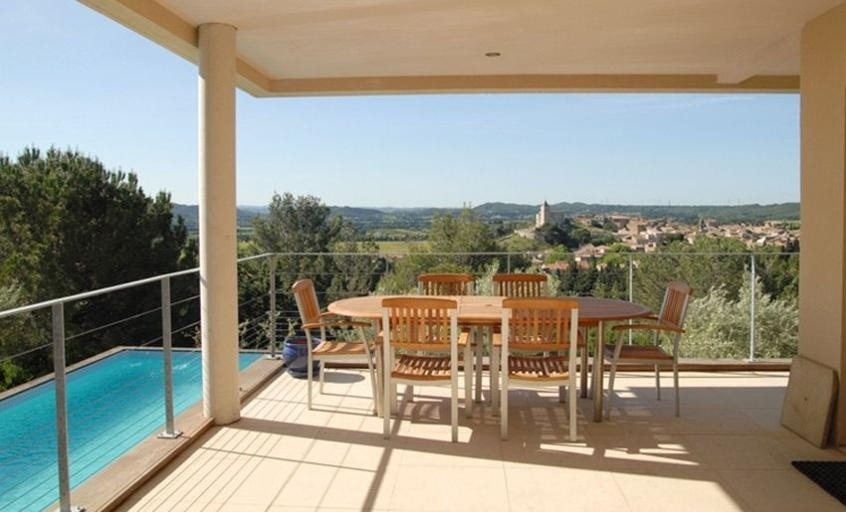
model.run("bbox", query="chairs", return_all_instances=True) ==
[493,296,580,442]
[599,280,693,423]
[378,295,473,442]
[294,278,383,417]
[487,274,553,414]
[406,275,483,404]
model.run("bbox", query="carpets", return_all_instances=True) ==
[791,461,846,506]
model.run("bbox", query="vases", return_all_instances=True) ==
[282,336,320,378]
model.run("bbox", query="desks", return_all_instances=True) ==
[328,294,651,423]
